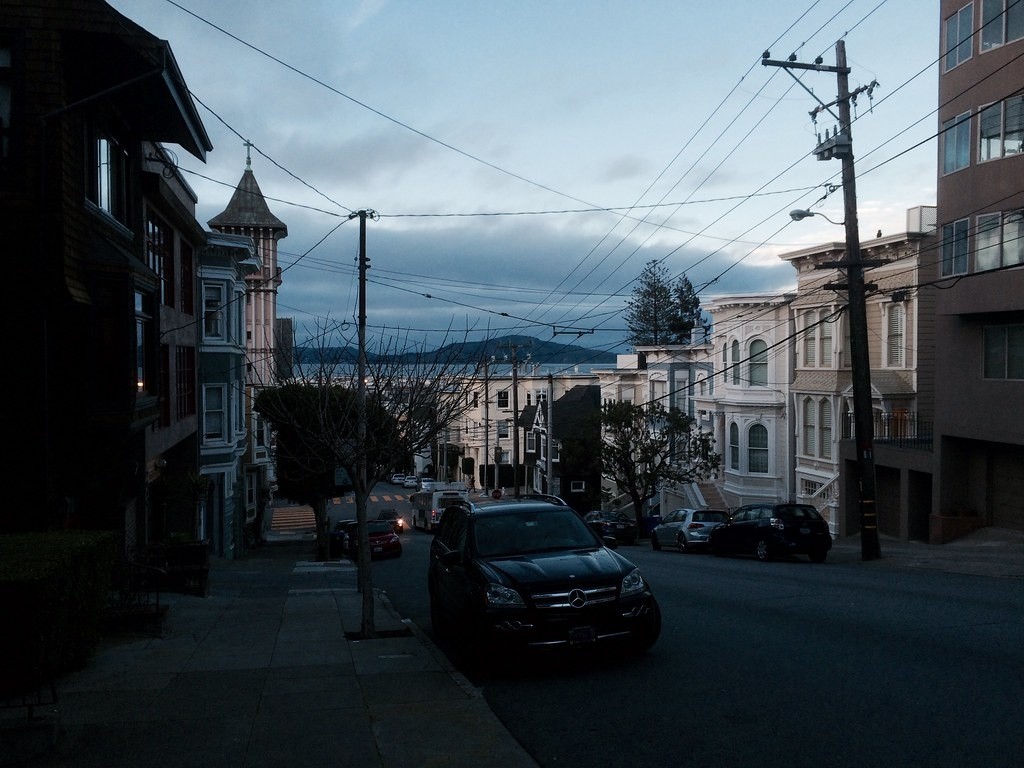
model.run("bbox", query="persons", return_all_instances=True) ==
[467,477,477,493]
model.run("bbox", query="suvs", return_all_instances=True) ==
[377,509,404,535]
[429,491,663,666]
[710,503,831,563]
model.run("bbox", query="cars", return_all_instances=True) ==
[404,476,418,488]
[584,511,638,546]
[416,478,434,492]
[334,520,354,533]
[349,520,402,560]
[652,509,728,553]
[386,474,405,484]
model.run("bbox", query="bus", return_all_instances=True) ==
[410,489,465,531]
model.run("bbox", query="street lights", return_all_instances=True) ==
[791,210,879,563]
